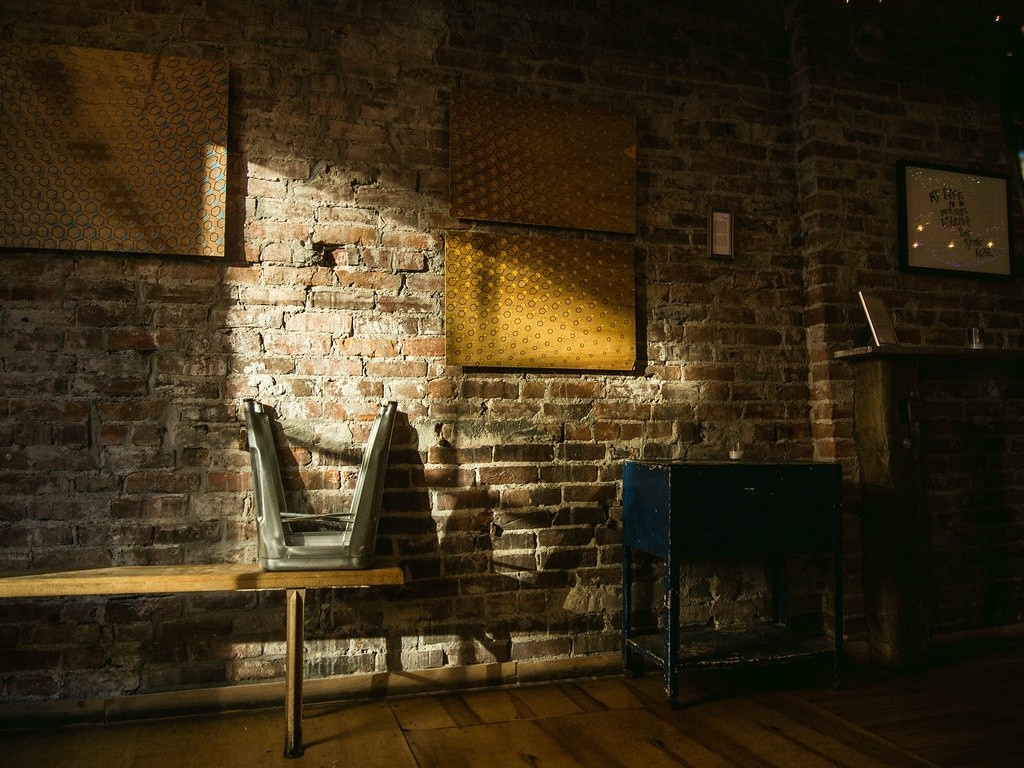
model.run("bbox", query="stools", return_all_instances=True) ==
[243,397,398,571]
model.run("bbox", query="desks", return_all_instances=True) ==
[0,564,405,758]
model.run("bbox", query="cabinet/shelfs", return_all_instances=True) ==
[620,460,843,701]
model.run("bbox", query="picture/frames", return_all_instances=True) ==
[897,161,1016,283]
[859,291,901,348]
